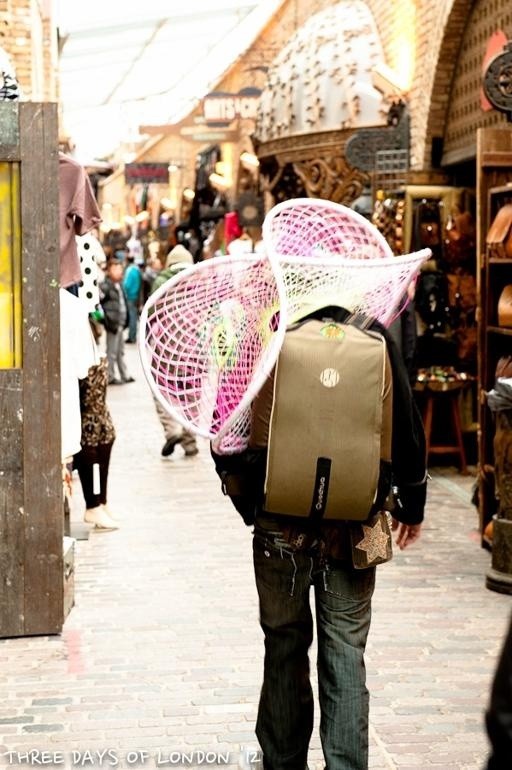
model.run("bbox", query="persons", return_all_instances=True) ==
[210,302,426,769]
[58,166,344,519]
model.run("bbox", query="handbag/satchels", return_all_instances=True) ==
[416,198,477,367]
[485,202,512,412]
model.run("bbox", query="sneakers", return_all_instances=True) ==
[163,435,198,458]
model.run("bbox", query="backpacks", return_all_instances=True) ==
[249,317,393,524]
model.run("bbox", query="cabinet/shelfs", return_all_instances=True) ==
[473,124,512,556]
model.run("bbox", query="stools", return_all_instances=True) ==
[422,378,473,478]
